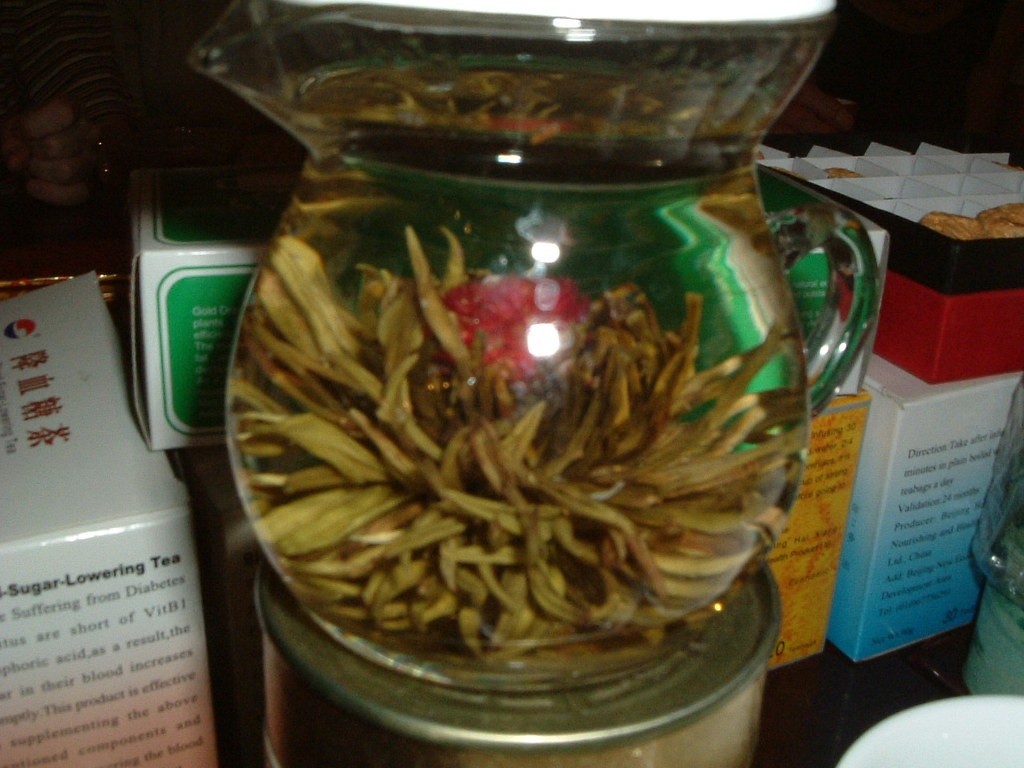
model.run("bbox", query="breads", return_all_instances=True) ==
[919,202,1024,240]
[822,168,862,178]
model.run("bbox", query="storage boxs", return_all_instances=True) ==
[123,180,890,458]
[0,267,220,768]
[752,390,879,669]
[753,136,1024,385]
[837,350,1024,659]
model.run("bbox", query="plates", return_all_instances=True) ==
[835,693,1023,767]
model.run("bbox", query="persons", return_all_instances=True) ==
[765,0,1024,135]
[0,0,137,210]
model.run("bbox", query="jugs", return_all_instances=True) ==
[188,1,882,692]
